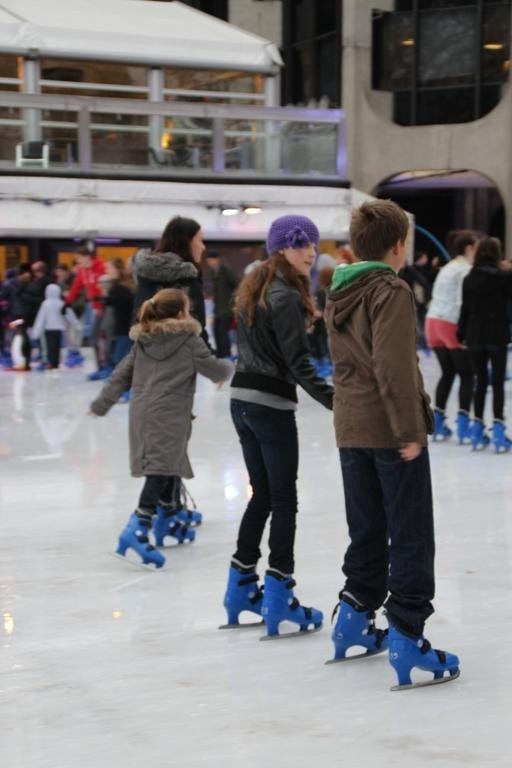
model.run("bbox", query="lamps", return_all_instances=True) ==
[243,205,263,215]
[218,204,239,216]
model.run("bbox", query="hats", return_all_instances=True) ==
[267,215,320,255]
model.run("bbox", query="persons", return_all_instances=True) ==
[398,259,416,291]
[63,260,78,286]
[129,217,220,525]
[53,264,75,289]
[324,201,461,690]
[205,251,239,358]
[218,215,335,641]
[10,268,42,371]
[30,260,52,292]
[32,284,84,370]
[423,230,480,443]
[93,257,131,403]
[0,269,16,301]
[312,266,333,366]
[59,248,120,381]
[412,253,431,332]
[87,287,235,573]
[428,255,442,283]
[454,235,512,454]
[334,239,361,265]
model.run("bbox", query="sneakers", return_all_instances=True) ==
[388,618,460,672]
[219,560,266,614]
[326,596,388,648]
[153,504,195,540]
[457,416,472,438]
[493,424,512,447]
[111,511,167,564]
[175,506,201,527]
[259,570,323,624]
[430,413,451,436]
[470,422,489,445]
